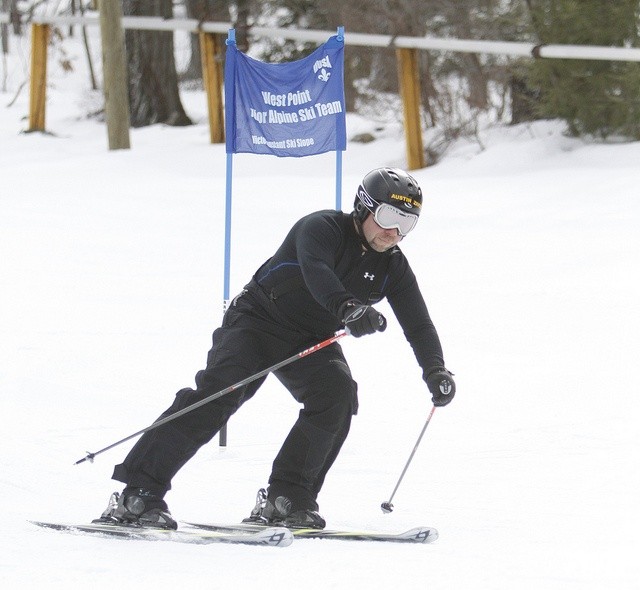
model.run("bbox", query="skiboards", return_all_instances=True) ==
[31,515,440,550]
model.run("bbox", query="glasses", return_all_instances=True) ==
[357,185,418,236]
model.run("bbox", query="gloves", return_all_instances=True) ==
[422,365,456,406]
[338,298,387,337]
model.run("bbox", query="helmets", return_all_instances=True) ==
[353,168,422,241]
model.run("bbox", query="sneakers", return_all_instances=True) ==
[264,488,325,529]
[117,487,178,530]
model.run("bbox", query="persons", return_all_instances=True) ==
[111,168,456,530]
